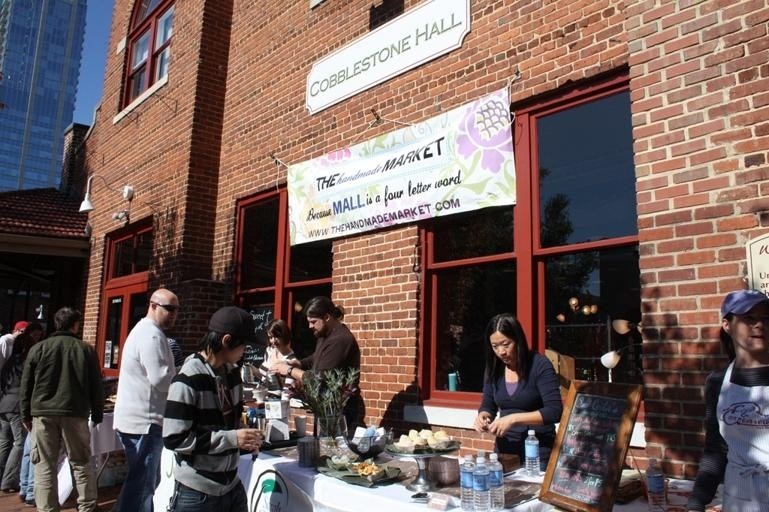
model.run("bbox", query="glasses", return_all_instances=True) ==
[150,299,182,311]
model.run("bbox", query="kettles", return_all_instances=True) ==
[239,365,254,382]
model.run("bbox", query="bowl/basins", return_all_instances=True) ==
[424,457,461,486]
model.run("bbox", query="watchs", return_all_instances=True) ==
[287,367,295,377]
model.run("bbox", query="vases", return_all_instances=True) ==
[317,415,349,441]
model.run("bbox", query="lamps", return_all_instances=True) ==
[77,173,134,216]
[598,317,642,369]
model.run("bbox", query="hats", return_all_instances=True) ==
[14,321,29,330]
[721,288,768,318]
[209,306,268,349]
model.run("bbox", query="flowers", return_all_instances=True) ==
[287,364,361,439]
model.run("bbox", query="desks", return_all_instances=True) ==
[149,425,723,512]
[83,411,124,493]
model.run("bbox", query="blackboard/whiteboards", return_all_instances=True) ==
[243,303,274,368]
[539,380,643,512]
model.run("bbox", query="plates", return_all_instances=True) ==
[345,461,363,474]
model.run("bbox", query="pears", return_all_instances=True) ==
[399,429,451,450]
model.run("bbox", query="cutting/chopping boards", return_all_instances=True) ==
[454,448,519,473]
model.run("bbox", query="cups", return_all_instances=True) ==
[253,388,268,403]
[297,436,320,468]
[242,388,253,400]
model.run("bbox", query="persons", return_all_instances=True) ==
[19,306,105,512]
[474,313,562,472]
[162,306,264,512]
[113,288,179,512]
[686,289,769,512]
[0,320,43,508]
[258,318,301,397]
[270,297,365,429]
[167,338,185,367]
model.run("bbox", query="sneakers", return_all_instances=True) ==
[0,481,99,512]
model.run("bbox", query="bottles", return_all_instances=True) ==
[256,412,266,431]
[460,448,505,510]
[524,430,541,477]
[247,408,255,427]
[448,369,458,391]
[293,415,306,437]
[646,459,669,510]
